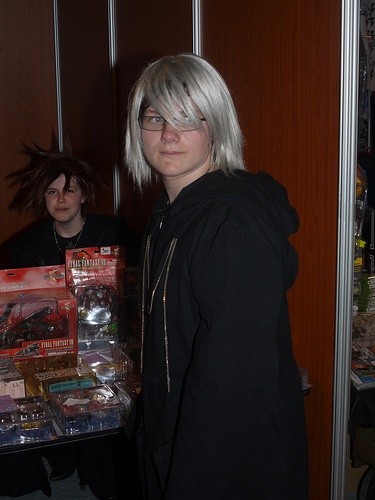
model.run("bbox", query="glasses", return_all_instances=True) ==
[138,116,206,131]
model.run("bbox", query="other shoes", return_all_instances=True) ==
[48,465,66,482]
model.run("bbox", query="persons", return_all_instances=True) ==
[124,54,312,500]
[0,142,119,269]
